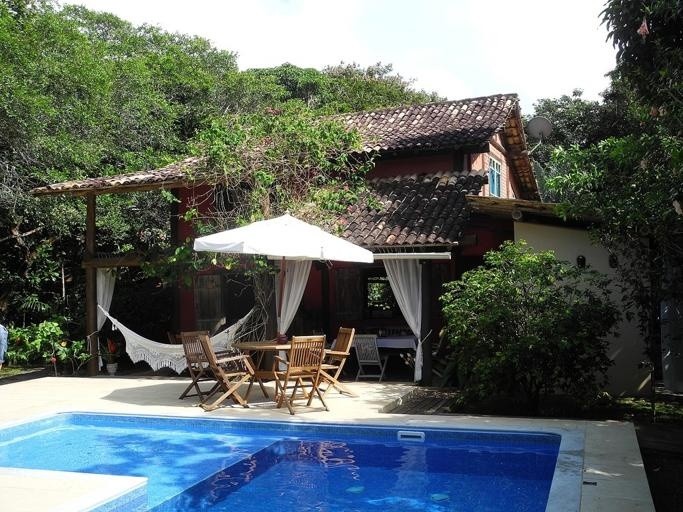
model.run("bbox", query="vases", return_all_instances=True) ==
[105,363,118,376]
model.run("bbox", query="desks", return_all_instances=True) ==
[330,333,417,378]
[230,340,322,404]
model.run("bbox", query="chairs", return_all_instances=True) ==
[192,329,254,412]
[273,334,328,414]
[320,326,356,397]
[351,332,390,384]
[176,329,240,401]
[375,326,401,337]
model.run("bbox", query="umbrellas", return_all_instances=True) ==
[193,208,373,398]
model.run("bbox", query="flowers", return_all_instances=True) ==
[99,339,123,362]
